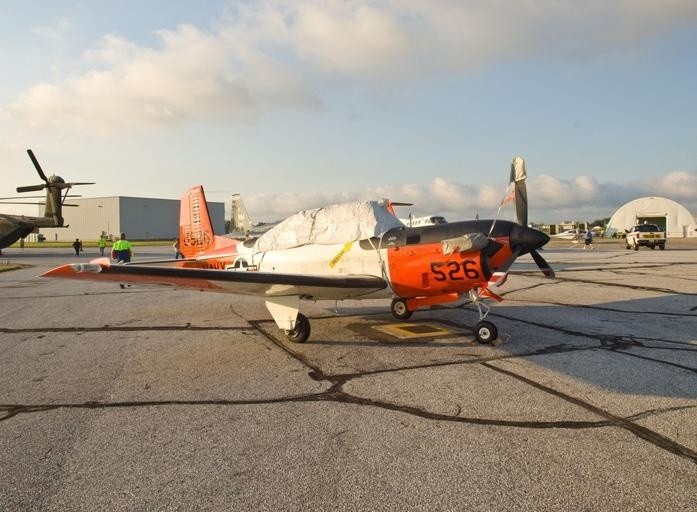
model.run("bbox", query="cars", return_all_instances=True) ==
[555,229,596,242]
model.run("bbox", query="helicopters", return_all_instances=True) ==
[1,148,96,252]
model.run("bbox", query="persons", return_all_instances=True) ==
[173,237,184,259]
[98,235,106,257]
[600,228,605,238]
[111,232,132,288]
[72,238,81,256]
[20,238,24,248]
[583,229,594,251]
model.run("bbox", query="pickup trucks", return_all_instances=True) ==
[625,224,666,250]
